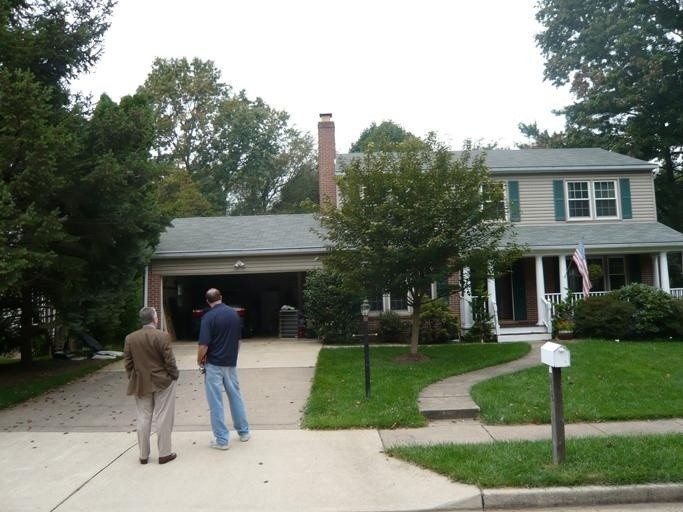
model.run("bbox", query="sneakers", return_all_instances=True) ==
[241,430,251,441]
[207,440,230,449]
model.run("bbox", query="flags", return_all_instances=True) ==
[572,240,592,299]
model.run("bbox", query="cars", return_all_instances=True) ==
[191,293,258,339]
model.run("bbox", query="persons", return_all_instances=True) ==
[123,306,180,465]
[195,288,251,449]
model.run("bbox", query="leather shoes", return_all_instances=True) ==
[158,453,176,464]
[140,459,147,464]
